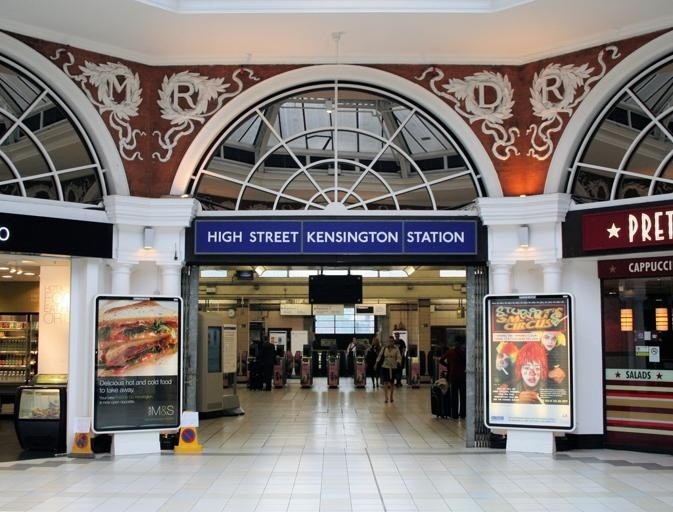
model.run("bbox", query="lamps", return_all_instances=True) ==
[325,95,333,114]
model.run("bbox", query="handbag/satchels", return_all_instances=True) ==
[376,353,385,367]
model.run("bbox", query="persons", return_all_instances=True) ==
[493,329,569,405]
[347,332,406,403]
[261,334,276,391]
[439,337,467,420]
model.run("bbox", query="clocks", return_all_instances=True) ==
[227,308,235,318]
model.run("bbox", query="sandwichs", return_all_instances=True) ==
[95,300,178,376]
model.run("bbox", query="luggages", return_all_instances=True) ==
[431,370,449,416]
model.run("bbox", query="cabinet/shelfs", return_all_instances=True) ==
[0,311,40,410]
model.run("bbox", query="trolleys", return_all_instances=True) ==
[242,359,264,391]
